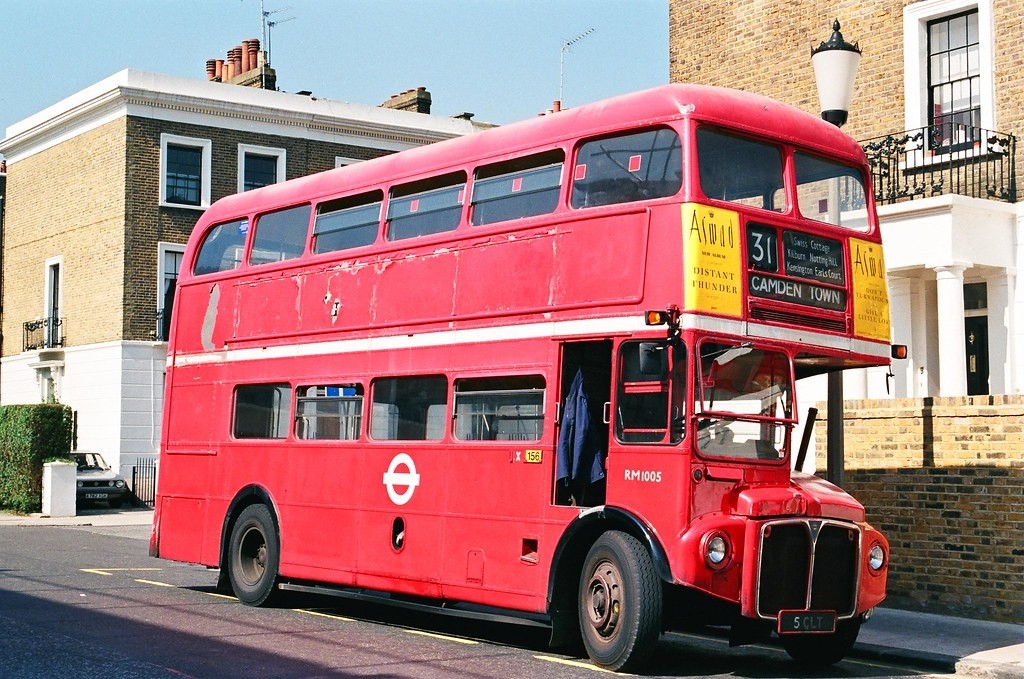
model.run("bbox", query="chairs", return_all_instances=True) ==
[368,402,543,445]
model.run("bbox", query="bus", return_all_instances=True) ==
[150,83,908,671]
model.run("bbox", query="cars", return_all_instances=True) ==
[70,451,126,509]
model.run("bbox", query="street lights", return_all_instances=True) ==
[811,18,862,491]
